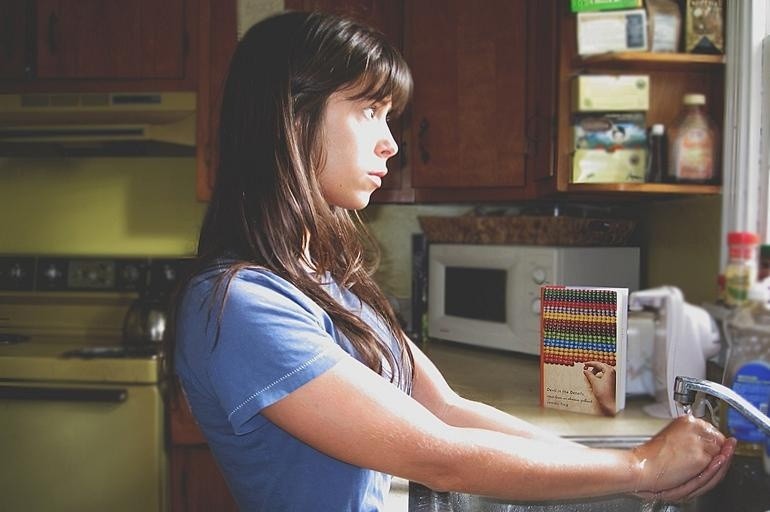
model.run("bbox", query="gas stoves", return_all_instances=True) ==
[1,330,164,384]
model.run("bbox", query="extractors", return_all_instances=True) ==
[2,92,201,154]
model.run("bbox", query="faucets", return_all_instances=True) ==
[673,375,766,433]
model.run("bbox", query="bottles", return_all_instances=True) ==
[718,283,769,458]
[722,231,760,308]
[754,242,769,308]
[667,91,724,184]
[646,123,668,183]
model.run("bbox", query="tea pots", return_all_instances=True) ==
[119,285,170,353]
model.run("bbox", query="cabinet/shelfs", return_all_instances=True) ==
[2,1,197,98]
[199,1,554,204]
[558,1,724,203]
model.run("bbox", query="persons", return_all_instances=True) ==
[168,9,741,509]
[582,361,616,416]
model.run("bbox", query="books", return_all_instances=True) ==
[569,72,652,189]
[538,282,632,418]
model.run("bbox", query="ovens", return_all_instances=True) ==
[1,377,167,512]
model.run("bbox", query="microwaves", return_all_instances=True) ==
[424,239,641,358]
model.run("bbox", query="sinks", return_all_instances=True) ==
[408,433,766,512]
